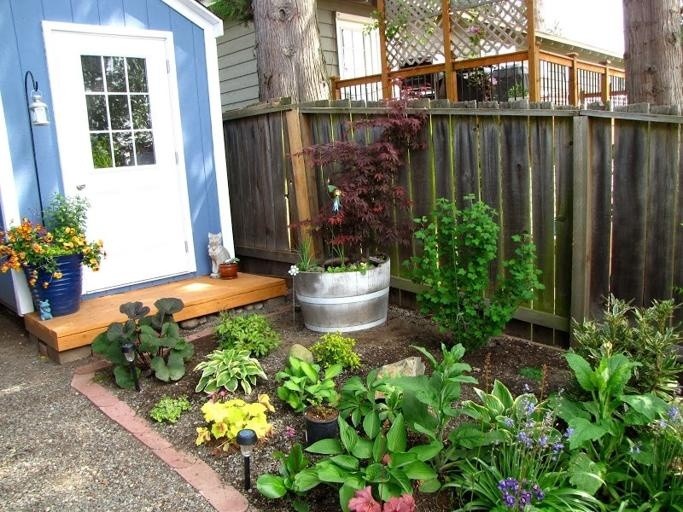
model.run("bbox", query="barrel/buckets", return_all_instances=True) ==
[293,252,391,331]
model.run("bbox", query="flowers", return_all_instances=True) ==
[0,182,106,290]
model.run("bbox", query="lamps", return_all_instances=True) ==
[23,70,51,226]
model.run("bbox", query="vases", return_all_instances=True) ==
[23,253,84,318]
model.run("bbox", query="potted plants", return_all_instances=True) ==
[280,79,437,335]
[219,257,240,280]
[284,355,346,449]
